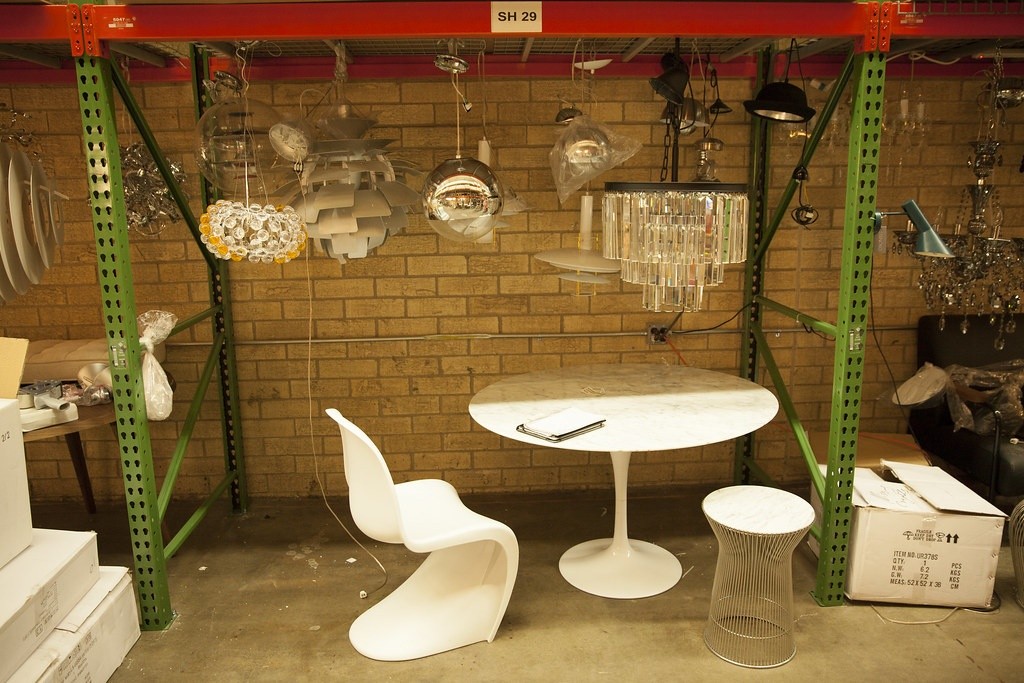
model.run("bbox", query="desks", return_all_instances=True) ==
[23,403,177,558]
[469,363,780,599]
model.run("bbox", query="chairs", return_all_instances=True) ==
[324,406,519,661]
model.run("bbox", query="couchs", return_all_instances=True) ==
[906,314,1024,509]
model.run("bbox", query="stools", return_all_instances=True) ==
[701,485,815,670]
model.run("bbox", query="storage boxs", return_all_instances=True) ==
[0,336,142,683]
[808,430,1010,610]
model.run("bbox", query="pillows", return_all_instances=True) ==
[19,339,168,384]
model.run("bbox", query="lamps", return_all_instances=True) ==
[193,40,424,265]
[783,54,928,184]
[875,199,955,259]
[422,38,528,243]
[600,41,748,314]
[535,182,621,297]
[555,47,612,165]
[744,82,817,123]
[971,47,1024,58]
[0,100,190,305]
[890,46,1024,349]
